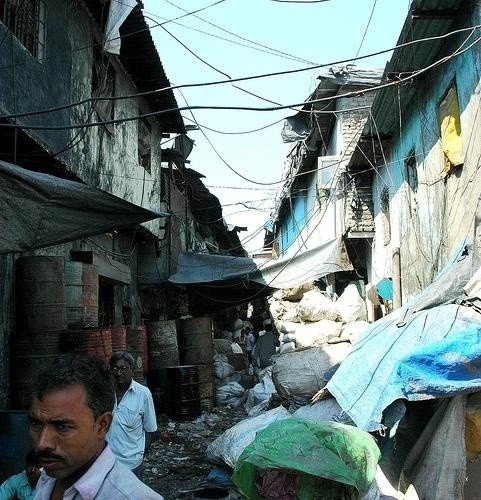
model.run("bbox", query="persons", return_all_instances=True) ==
[237,329,246,346]
[102,350,159,482]
[238,327,256,368]
[30,351,168,500]
[0,451,44,500]
[252,323,280,372]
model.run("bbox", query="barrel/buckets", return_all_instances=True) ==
[10,256,64,334]
[4,332,64,409]
[57,326,114,369]
[162,364,201,417]
[125,323,148,376]
[62,260,84,326]
[108,325,127,350]
[180,317,212,365]
[195,365,215,411]
[84,263,100,327]
[132,374,148,387]
[142,319,180,368]
[149,365,163,418]
[1,410,35,483]
